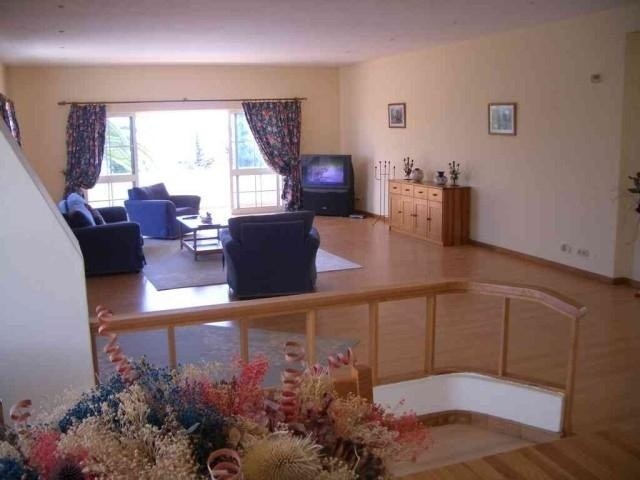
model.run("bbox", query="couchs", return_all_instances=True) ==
[58,200,146,274]
[124,195,201,238]
[219,211,320,300]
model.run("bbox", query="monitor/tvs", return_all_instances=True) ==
[301,154,353,188]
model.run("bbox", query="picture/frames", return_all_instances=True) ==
[388,103,406,128]
[489,104,515,135]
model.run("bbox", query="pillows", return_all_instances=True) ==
[127,184,170,200]
[67,194,86,208]
[68,205,95,227]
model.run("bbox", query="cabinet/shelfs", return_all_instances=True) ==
[388,178,470,247]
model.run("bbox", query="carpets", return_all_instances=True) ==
[139,226,364,291]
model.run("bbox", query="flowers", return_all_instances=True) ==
[403,157,413,175]
[449,160,460,179]
